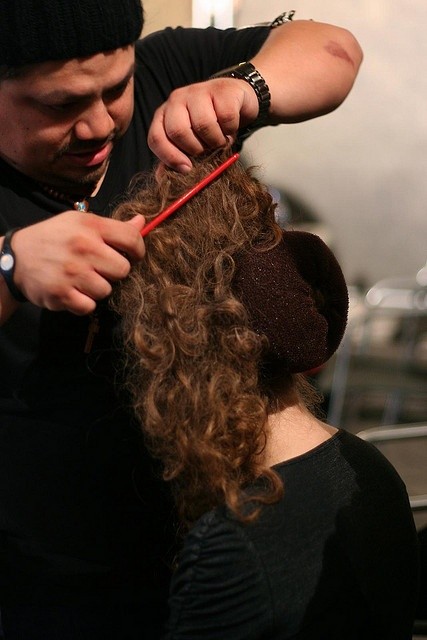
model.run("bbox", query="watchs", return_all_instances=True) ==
[0,227,27,306]
[208,62,271,123]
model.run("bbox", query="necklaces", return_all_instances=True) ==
[28,178,99,212]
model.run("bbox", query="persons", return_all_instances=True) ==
[100,146,416,635]
[1,0,364,333]
